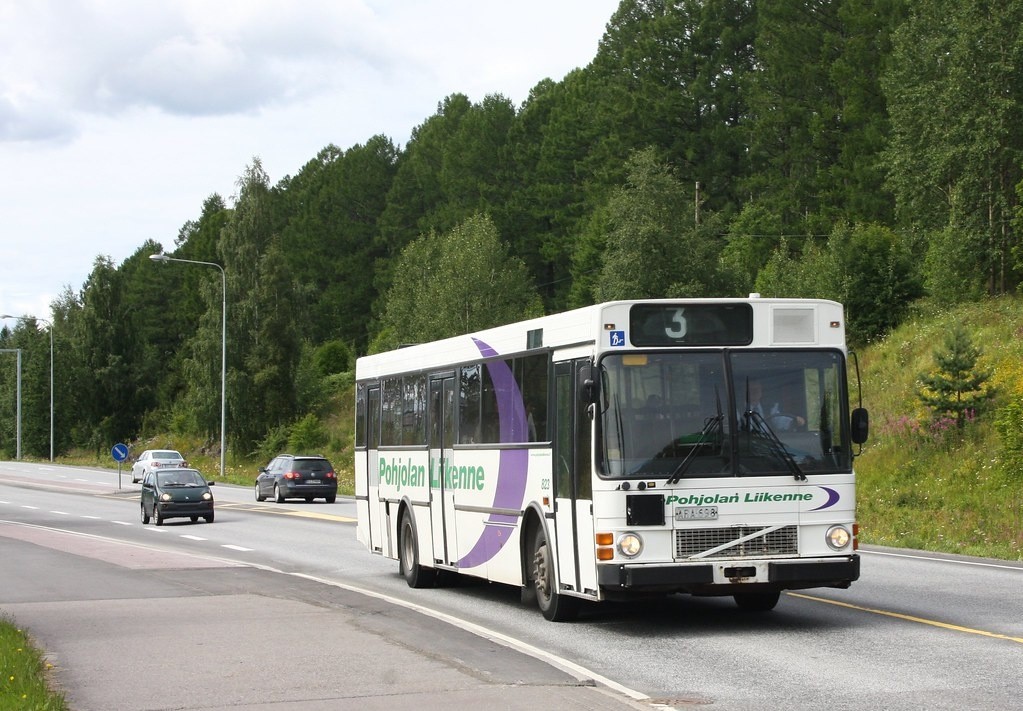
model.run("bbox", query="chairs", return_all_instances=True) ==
[621,404,703,447]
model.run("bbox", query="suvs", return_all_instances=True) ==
[252,452,338,504]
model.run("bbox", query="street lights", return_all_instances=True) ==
[0,314,55,463]
[150,253,226,478]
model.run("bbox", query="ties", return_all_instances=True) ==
[753,406,760,432]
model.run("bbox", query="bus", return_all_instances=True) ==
[353,293,872,624]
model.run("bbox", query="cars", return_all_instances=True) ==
[130,448,188,484]
[139,467,215,526]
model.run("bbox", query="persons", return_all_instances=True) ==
[178,474,190,483]
[736,374,805,433]
[459,393,546,443]
[638,394,670,421]
